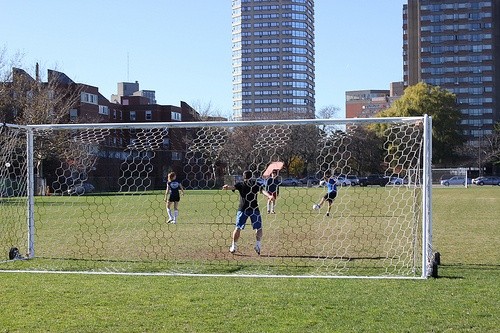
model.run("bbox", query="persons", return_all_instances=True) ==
[164,172,185,223]
[222,171,275,254]
[318,176,338,216]
[264,169,280,213]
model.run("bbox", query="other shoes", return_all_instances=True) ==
[326,212,329,218]
[267,211,270,214]
[167,219,173,223]
[171,221,175,223]
[271,212,275,214]
[230,247,235,253]
[254,247,260,254]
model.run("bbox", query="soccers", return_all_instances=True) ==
[312,205,319,210]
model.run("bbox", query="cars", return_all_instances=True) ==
[280,177,299,186]
[319,174,404,187]
[299,176,320,185]
[255,178,265,188]
[67,183,95,194]
[471,176,500,186]
[441,176,470,186]
